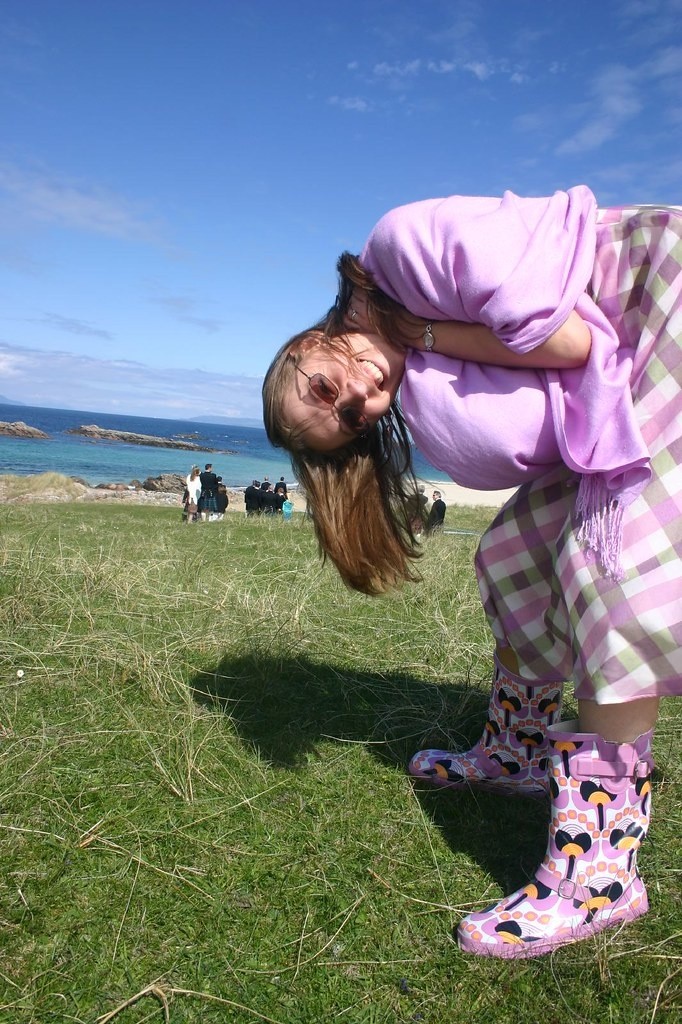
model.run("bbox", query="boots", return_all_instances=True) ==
[408,671,563,796]
[458,731,653,959]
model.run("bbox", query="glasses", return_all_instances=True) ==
[295,362,371,440]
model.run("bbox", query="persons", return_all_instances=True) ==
[262,192,682,961]
[245,477,294,522]
[406,485,446,535]
[182,463,228,524]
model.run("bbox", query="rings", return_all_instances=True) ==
[351,309,357,320]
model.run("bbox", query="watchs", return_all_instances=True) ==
[423,320,434,352]
